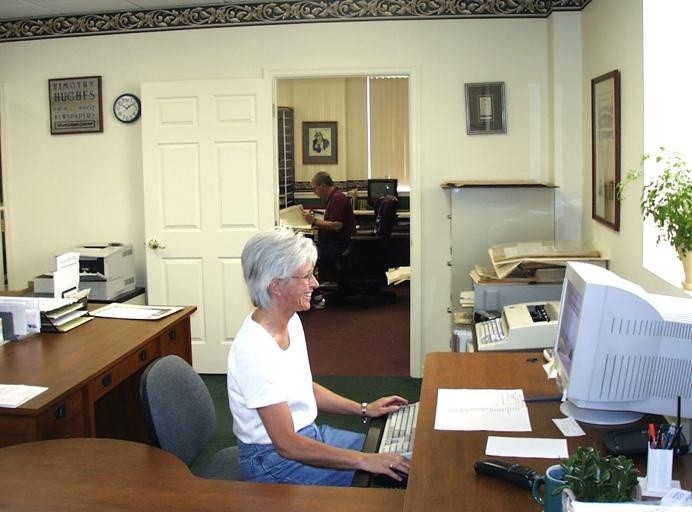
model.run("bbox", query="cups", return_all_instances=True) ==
[646,441,673,491]
[533,464,571,511]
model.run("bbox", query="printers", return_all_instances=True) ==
[54,241,137,301]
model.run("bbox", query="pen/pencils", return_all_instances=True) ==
[648,423,684,449]
[522,397,568,402]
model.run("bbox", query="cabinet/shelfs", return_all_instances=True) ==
[440,179,556,351]
[451,239,611,352]
[22,286,146,306]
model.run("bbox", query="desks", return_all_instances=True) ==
[279,206,319,310]
[1,290,196,447]
[404,349,691,512]
[0,437,405,511]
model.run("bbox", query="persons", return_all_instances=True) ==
[227,227,410,486]
[313,131,329,152]
[302,171,353,309]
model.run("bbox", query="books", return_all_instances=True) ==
[468,242,611,286]
[459,291,481,308]
[280,204,308,228]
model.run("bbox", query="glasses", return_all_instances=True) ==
[291,274,311,284]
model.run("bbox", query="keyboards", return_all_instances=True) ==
[378,401,419,460]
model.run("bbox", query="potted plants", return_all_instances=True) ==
[552,442,640,512]
[614,144,692,291]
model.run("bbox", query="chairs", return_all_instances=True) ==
[319,195,399,307]
[140,354,240,480]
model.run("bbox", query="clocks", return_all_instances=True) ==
[113,93,141,124]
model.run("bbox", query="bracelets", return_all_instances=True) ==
[312,218,317,227]
[360,403,368,425]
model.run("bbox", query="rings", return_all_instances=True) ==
[389,461,394,469]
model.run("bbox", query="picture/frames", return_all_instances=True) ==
[302,121,338,164]
[589,69,621,232]
[48,76,104,135]
[464,82,507,136]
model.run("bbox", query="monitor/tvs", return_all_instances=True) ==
[553,261,692,425]
[368,179,397,206]
